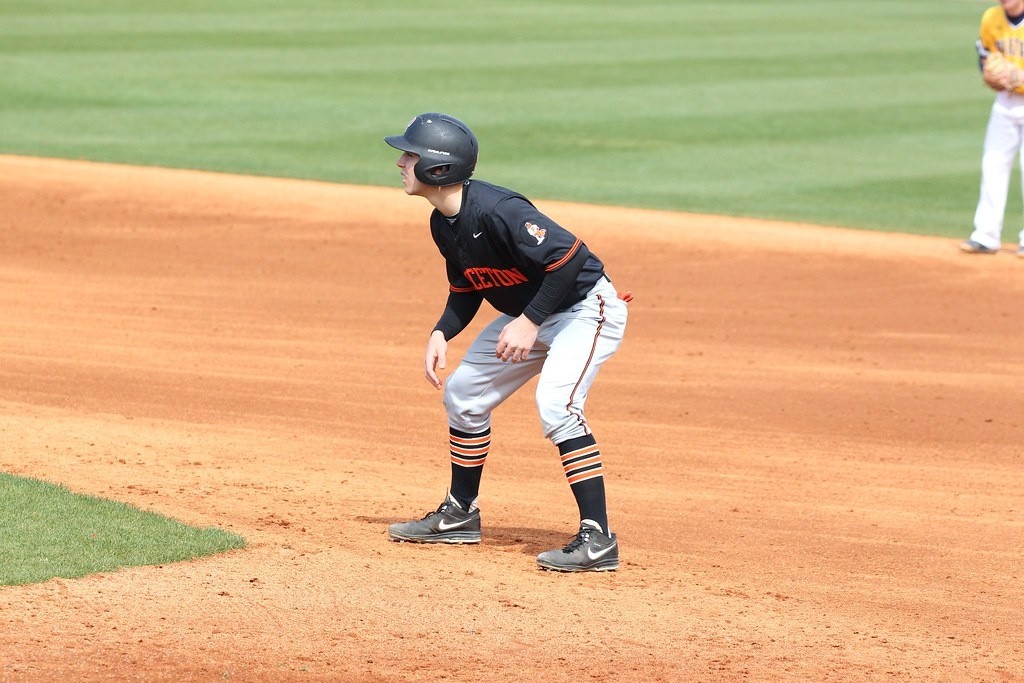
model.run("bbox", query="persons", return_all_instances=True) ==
[382,113,635,571]
[961,0,1024,257]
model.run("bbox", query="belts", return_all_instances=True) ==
[583,274,611,299]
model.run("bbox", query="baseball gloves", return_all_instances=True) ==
[981,52,1024,92]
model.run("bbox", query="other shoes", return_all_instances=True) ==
[961,241,996,254]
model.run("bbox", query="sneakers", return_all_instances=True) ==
[536,522,619,572]
[388,496,481,543]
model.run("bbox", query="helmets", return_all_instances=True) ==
[383,113,477,186]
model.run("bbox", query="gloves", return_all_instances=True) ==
[617,290,634,303]
